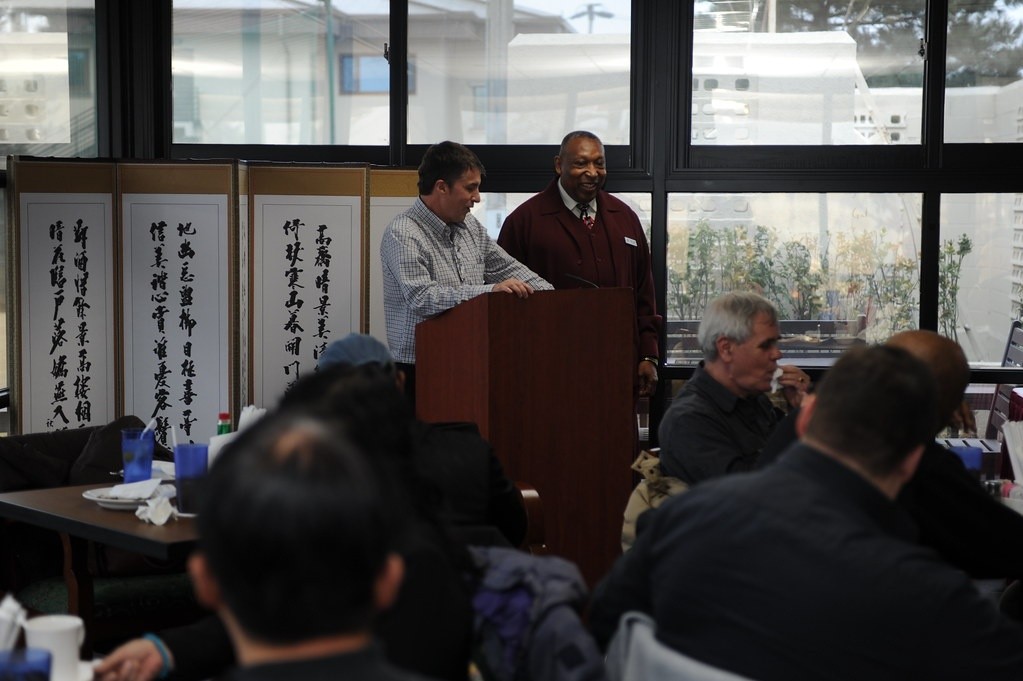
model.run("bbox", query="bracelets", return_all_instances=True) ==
[145,631,170,677]
[638,354,660,369]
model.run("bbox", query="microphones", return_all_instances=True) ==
[564,274,598,288]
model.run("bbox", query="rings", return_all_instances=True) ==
[799,378,804,383]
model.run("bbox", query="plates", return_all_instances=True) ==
[76,660,96,681]
[83,488,147,510]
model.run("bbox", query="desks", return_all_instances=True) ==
[0,479,203,660]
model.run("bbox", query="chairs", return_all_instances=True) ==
[936,318,1023,479]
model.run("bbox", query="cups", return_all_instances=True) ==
[984,480,1001,503]
[173,443,208,514]
[122,429,154,484]
[949,447,982,474]
[0,613,86,681]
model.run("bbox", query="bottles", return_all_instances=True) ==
[217,412,230,435]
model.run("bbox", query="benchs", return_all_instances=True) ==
[666,314,869,408]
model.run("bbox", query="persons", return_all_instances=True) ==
[315,332,528,544]
[584,343,1023,681]
[86,364,606,681]
[184,408,423,681]
[380,140,557,412]
[753,330,1023,580]
[657,297,814,482]
[496,130,663,482]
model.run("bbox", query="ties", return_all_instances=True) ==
[576,202,594,229]
